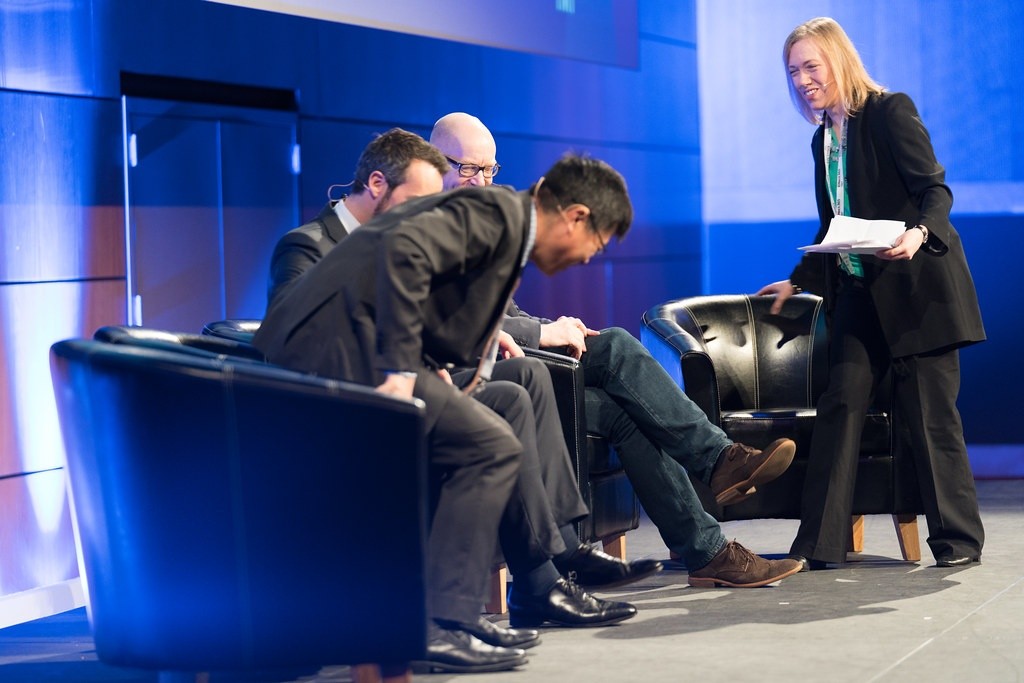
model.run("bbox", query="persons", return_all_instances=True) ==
[253,155,632,675]
[267,126,664,630]
[429,112,803,590]
[755,16,986,572]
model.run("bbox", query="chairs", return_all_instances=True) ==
[200,319,638,560]
[95,323,508,615]
[49,337,431,683]
[640,293,921,563]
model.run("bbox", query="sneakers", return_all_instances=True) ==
[687,538,803,587]
[669,550,682,561]
[709,437,797,506]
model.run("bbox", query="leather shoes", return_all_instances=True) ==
[505,571,638,627]
[433,614,542,650]
[935,555,974,567]
[784,554,827,572]
[551,538,664,589]
[409,627,529,673]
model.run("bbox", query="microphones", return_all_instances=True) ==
[823,79,835,87]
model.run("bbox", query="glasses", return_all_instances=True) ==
[578,201,609,256]
[445,156,501,178]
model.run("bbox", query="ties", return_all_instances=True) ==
[462,264,527,393]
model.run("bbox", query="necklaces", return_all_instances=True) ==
[830,117,848,162]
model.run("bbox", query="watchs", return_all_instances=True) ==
[915,226,928,243]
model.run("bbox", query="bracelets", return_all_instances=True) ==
[793,284,802,293]
